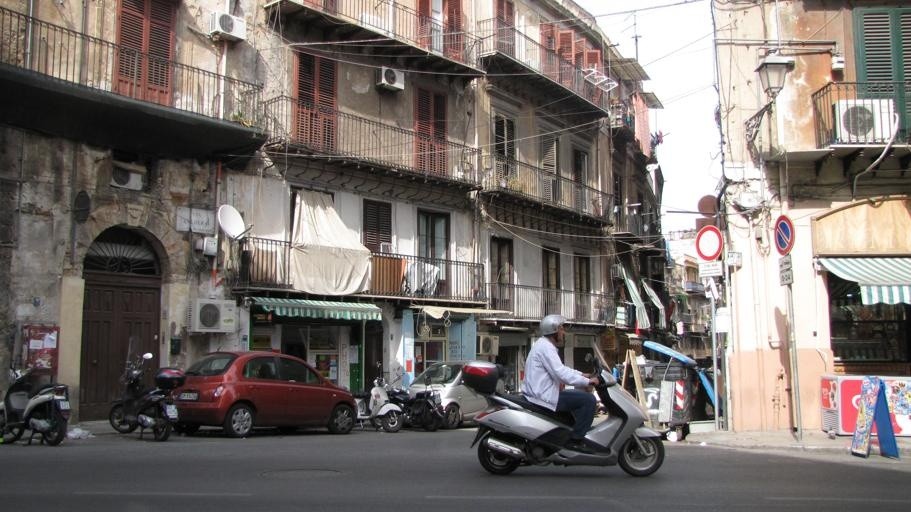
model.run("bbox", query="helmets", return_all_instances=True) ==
[538,314,569,336]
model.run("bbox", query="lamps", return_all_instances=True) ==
[752,47,795,104]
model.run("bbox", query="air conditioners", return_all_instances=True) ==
[375,65,404,91]
[476,335,499,356]
[110,161,144,191]
[186,297,236,335]
[835,99,896,144]
[210,11,247,41]
[609,264,623,279]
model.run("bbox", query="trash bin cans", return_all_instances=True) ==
[623,341,717,438]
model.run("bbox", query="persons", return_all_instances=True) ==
[521,313,604,454]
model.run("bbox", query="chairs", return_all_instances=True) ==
[222,359,277,379]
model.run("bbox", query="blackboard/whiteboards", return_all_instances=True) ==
[851,376,880,458]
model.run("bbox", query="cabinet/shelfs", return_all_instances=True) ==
[833,321,909,363]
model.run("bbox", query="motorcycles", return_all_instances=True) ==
[459,349,669,481]
[1,357,71,450]
[353,363,449,436]
[107,349,190,442]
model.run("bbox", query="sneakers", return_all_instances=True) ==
[565,437,597,454]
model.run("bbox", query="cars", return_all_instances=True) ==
[169,349,361,438]
[404,358,507,431]
